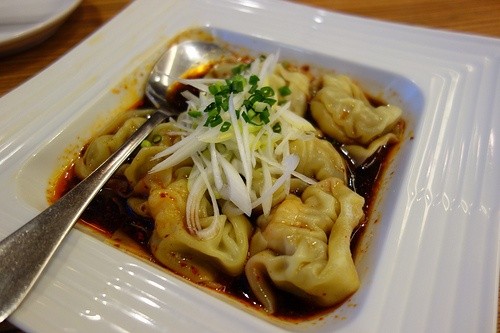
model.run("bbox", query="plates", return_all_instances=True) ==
[0,0,81,56]
[0,0,500,333]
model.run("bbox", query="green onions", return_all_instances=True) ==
[187,53,292,153]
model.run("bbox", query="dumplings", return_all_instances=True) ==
[126,179,253,291]
[245,177,366,312]
[276,66,310,117]
[310,71,405,164]
[74,108,190,183]
[251,136,348,205]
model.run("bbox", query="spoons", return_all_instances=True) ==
[1,41,226,324]
